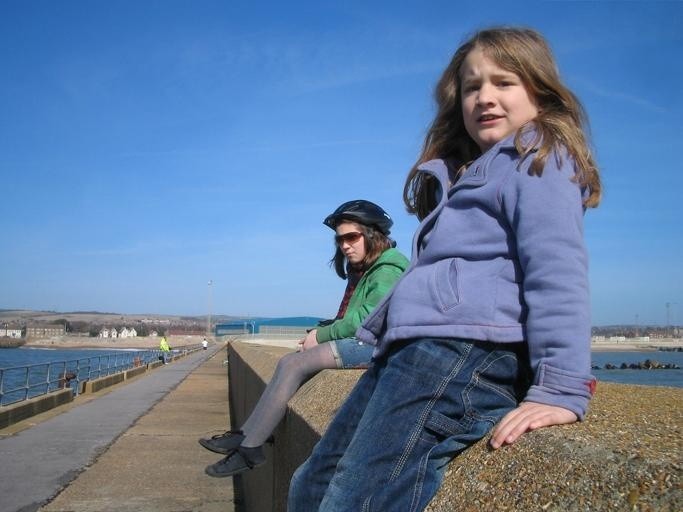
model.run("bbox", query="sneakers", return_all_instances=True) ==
[199,430,246,454]
[205,444,267,479]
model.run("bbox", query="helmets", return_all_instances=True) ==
[322,199,395,238]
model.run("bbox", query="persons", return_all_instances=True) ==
[199,200,410,478]
[202,339,208,354]
[286,27,597,512]
[160,336,169,366]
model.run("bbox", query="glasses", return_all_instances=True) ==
[334,231,370,245]
